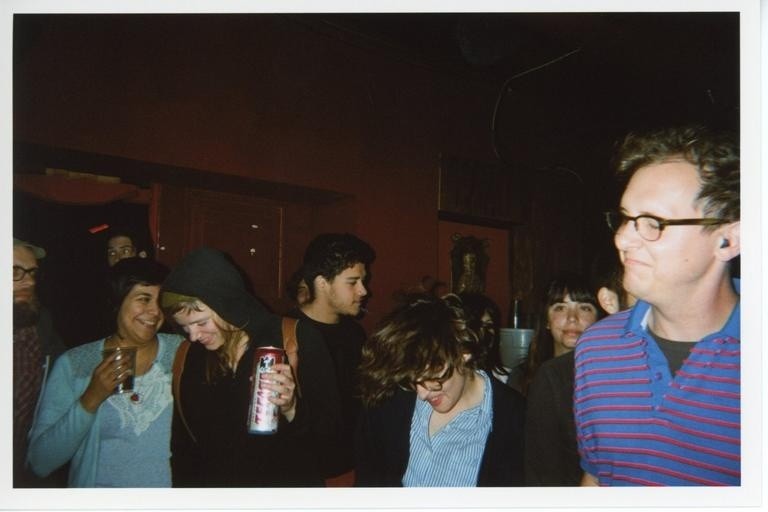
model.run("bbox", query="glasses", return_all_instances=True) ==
[605,210,734,242]
[13,265,39,283]
[395,360,454,393]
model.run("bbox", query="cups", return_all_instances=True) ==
[103,345,137,395]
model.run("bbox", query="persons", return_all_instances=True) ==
[501,266,606,396]
[571,126,739,484]
[517,246,636,487]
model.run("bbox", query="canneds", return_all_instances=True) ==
[246,346,288,436]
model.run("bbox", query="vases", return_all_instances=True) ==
[494,329,539,369]
